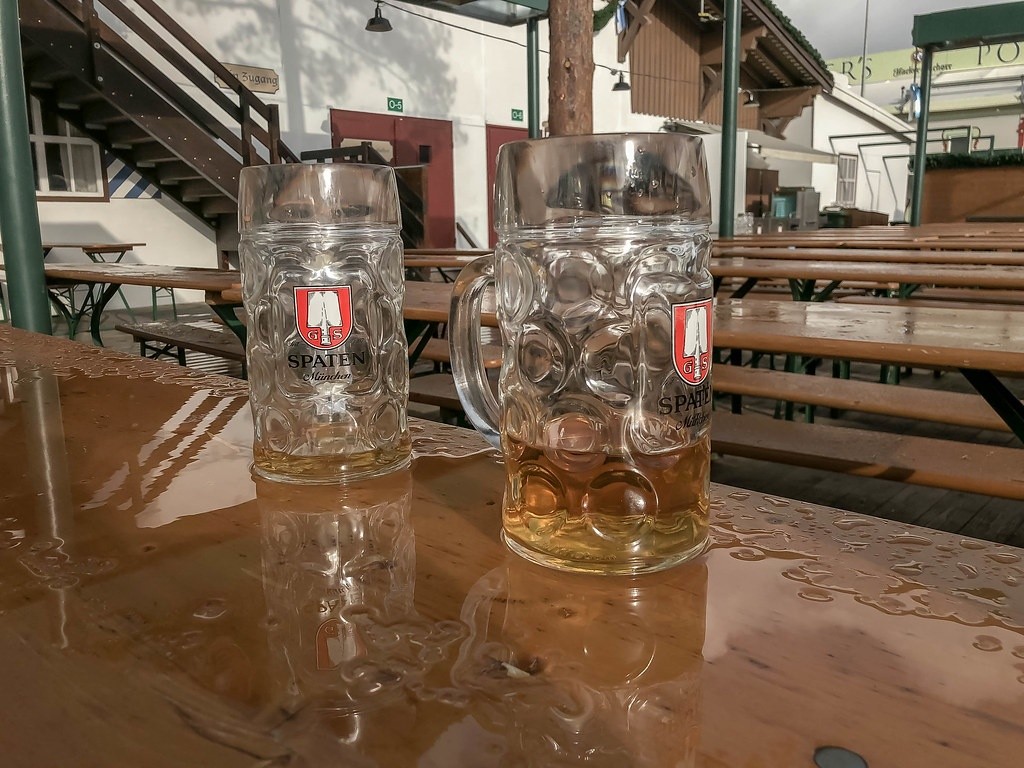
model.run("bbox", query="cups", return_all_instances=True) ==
[446,132,713,574]
[238,163,414,485]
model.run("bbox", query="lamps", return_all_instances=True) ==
[742,88,760,109]
[364,3,393,32]
[610,72,632,93]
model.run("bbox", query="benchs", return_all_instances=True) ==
[836,294,1024,312]
[912,288,1024,304]
[408,335,503,370]
[723,278,900,295]
[213,315,246,327]
[711,363,1012,432]
[116,317,247,366]
[718,292,794,301]
[407,369,499,425]
[706,406,1024,500]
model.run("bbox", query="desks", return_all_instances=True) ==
[0,222,1024,768]
